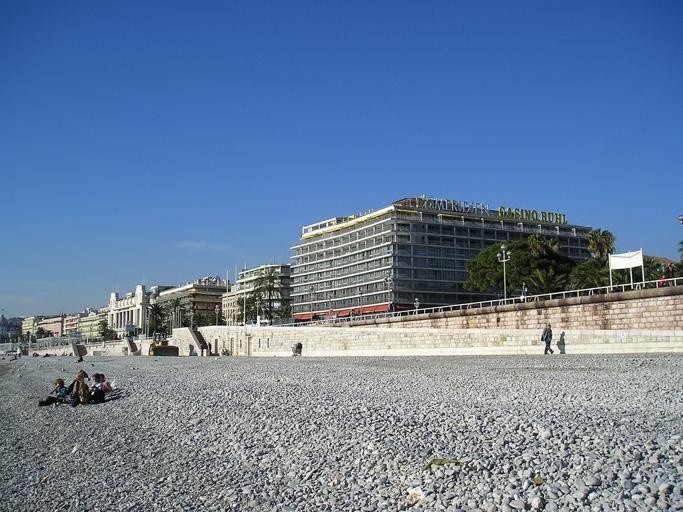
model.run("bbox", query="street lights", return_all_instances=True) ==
[306,285,315,321]
[255,296,260,324]
[383,270,394,311]
[215,304,220,325]
[496,243,511,304]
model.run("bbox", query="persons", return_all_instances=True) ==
[658,259,676,286]
[37,371,113,406]
[541,324,554,356]
